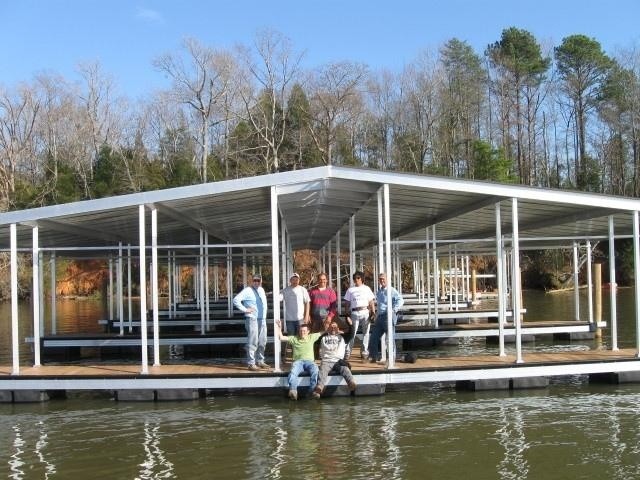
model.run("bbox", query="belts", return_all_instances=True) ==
[351,307,366,311]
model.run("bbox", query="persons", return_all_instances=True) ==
[313,315,357,399]
[231,273,271,372]
[279,273,310,336]
[277,317,330,400]
[368,272,404,364]
[343,271,376,360]
[308,274,338,330]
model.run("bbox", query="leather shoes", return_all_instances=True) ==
[312,390,320,399]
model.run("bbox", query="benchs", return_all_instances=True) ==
[330,286,606,363]
[25,283,284,364]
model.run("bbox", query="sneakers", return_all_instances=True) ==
[288,390,297,401]
[248,364,261,371]
[348,380,357,391]
[256,362,270,369]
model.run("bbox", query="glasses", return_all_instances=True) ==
[353,276,360,279]
[253,279,260,282]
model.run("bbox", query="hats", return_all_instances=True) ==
[378,273,386,279]
[290,273,299,279]
